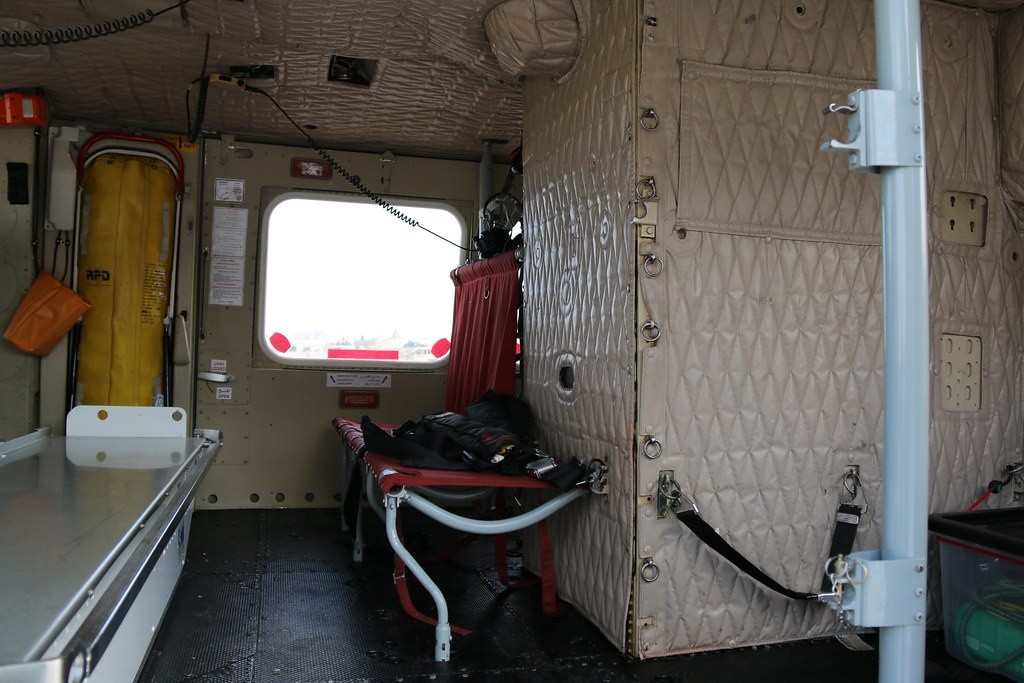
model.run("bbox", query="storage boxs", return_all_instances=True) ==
[928,505,1024,683]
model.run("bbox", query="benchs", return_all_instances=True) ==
[332,415,598,663]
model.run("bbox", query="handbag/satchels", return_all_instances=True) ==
[3,269,92,357]
